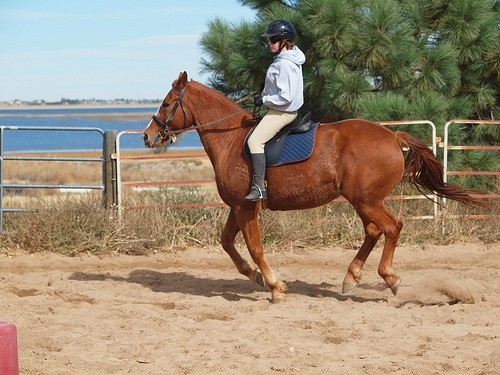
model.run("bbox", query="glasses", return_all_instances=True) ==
[269,37,280,44]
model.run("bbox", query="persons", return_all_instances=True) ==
[243,20,305,201]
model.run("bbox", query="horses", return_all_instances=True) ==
[143,71,495,305]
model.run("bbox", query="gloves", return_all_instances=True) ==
[254,97,263,106]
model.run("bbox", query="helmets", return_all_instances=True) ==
[262,20,296,38]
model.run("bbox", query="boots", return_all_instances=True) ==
[246,154,267,199]
